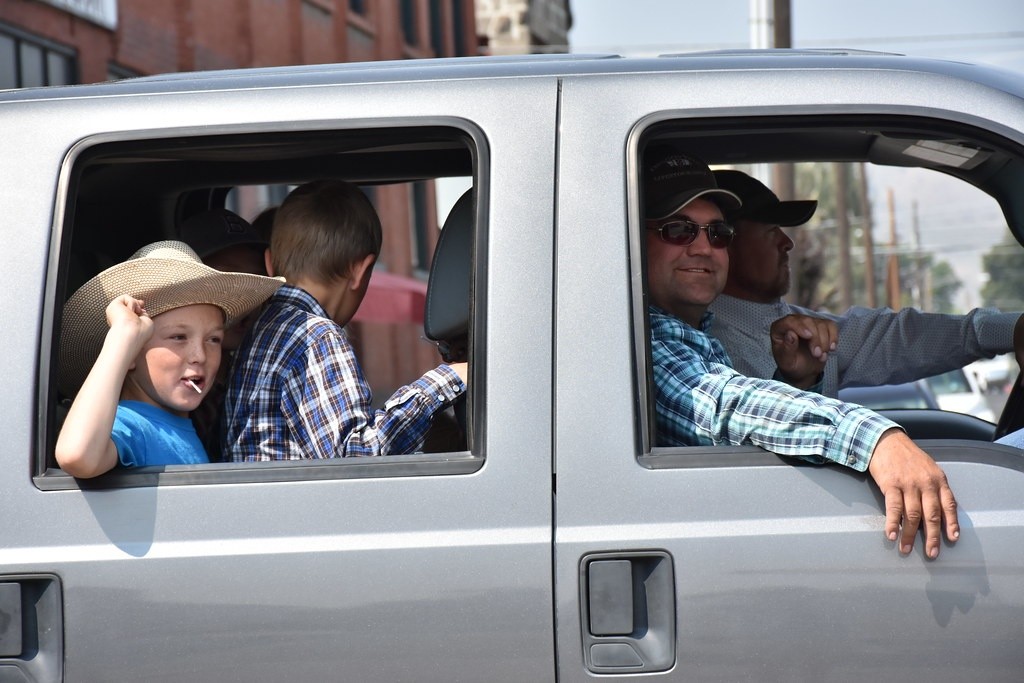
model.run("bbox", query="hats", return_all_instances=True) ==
[59,240,286,387]
[637,154,742,223]
[710,170,817,226]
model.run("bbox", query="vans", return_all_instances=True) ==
[0,52,1024,683]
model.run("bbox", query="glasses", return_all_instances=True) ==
[646,219,737,248]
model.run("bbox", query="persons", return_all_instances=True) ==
[632,149,961,559]
[54,177,469,481]
[701,169,1024,402]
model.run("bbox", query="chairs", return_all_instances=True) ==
[424,187,474,452]
